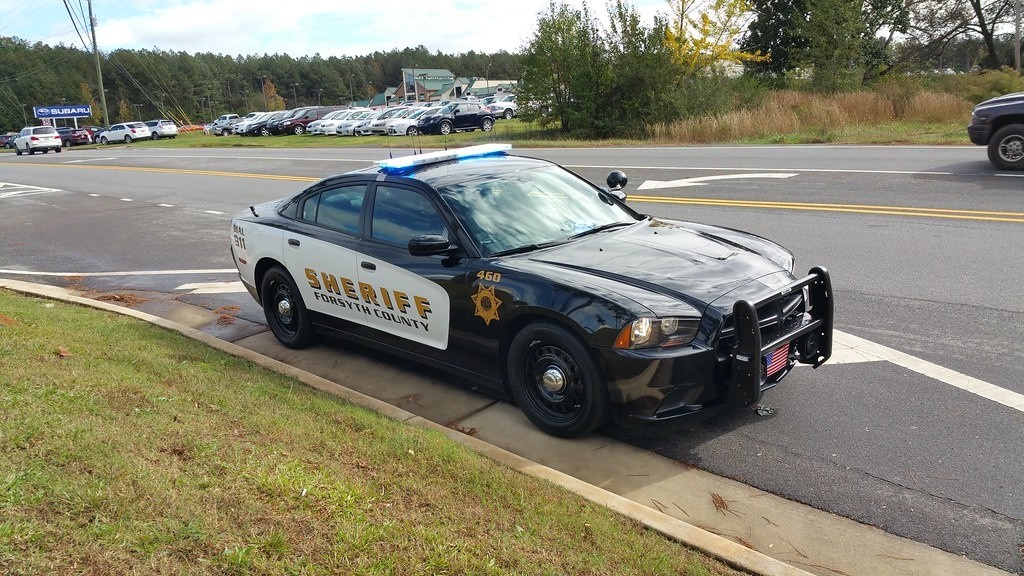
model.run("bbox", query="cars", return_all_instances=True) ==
[203,91,553,136]
[93,125,111,143]
[0,135,12,147]
[5,134,17,149]
[54,126,73,132]
[228,145,835,441]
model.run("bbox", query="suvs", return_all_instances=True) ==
[145,119,179,140]
[100,121,151,144]
[967,91,1024,170]
[82,125,99,142]
[13,126,62,155]
[58,128,92,147]
